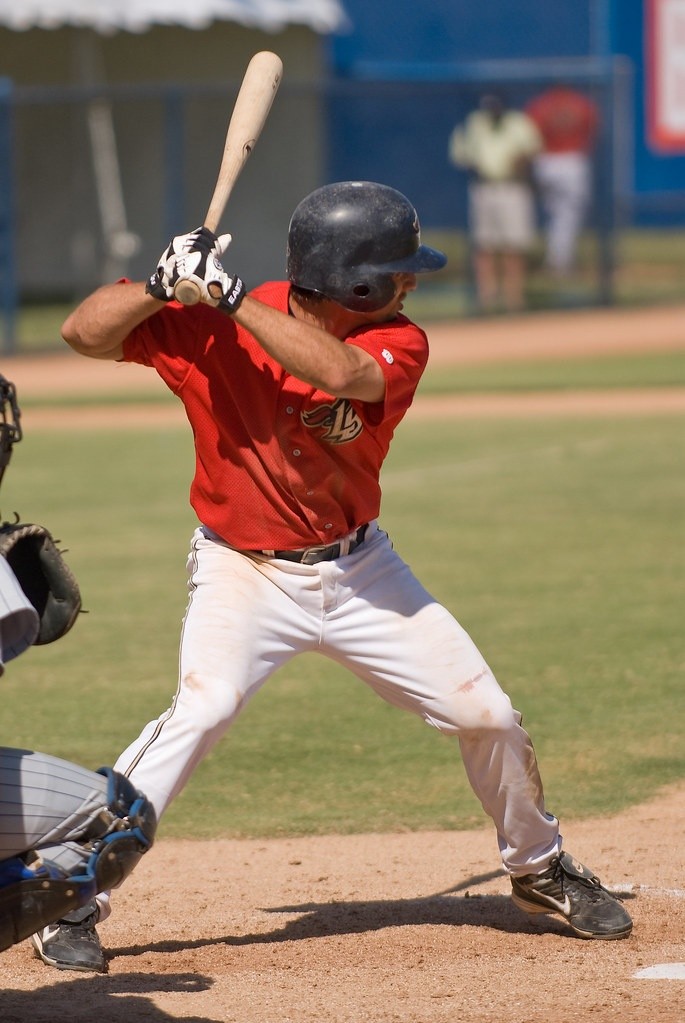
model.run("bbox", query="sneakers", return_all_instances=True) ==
[35,900,113,975]
[509,853,631,942]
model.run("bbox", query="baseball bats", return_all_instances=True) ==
[174,50,284,306]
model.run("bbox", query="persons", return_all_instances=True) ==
[444,79,599,310]
[30,180,637,972]
[0,376,156,946]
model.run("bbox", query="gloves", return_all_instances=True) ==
[145,227,245,312]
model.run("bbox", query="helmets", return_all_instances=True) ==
[286,182,452,315]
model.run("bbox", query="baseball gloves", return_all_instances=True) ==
[0,509,92,647]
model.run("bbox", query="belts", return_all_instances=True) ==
[252,524,369,565]
